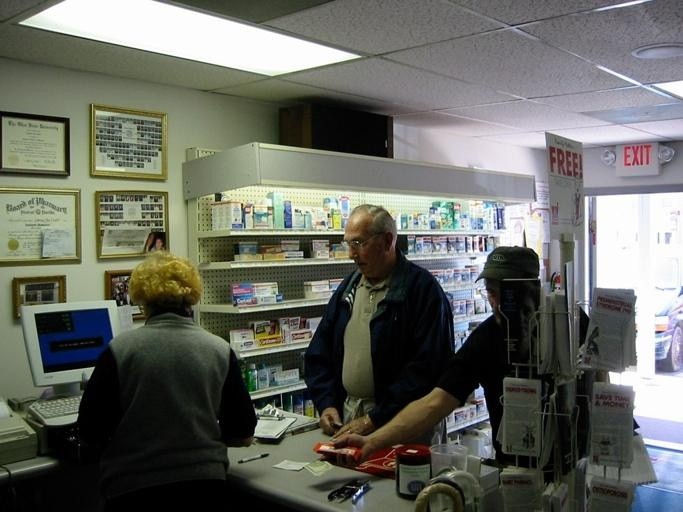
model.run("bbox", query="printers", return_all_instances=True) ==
[0,394,37,466]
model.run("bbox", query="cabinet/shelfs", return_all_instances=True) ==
[182,142,536,433]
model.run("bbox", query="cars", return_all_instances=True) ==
[653,275,682,375]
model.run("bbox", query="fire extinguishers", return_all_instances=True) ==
[551,272,561,293]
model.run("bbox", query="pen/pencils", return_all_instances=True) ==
[238,453,270,463]
[330,423,341,429]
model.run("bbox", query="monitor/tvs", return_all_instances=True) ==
[19,299,121,400]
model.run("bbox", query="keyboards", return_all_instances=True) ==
[29,393,85,426]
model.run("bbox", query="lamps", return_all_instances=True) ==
[600,142,675,179]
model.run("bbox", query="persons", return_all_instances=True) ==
[145,234,154,251]
[112,279,138,307]
[319,246,610,486]
[75,251,260,512]
[302,204,452,450]
[150,238,165,252]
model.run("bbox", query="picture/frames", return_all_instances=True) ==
[96,190,169,259]
[0,111,70,176]
[0,187,81,267]
[91,103,168,180]
[105,270,145,320]
[13,276,66,319]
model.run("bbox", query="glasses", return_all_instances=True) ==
[339,234,381,249]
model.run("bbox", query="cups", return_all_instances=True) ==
[428,442,468,479]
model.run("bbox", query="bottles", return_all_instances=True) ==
[295,209,304,227]
[247,362,257,393]
[256,363,269,391]
[394,445,430,501]
[283,199,292,228]
[239,357,247,388]
[255,198,274,230]
[304,210,312,228]
[253,390,321,420]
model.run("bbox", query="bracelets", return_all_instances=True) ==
[362,414,370,429]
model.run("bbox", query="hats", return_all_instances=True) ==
[473,245,540,283]
